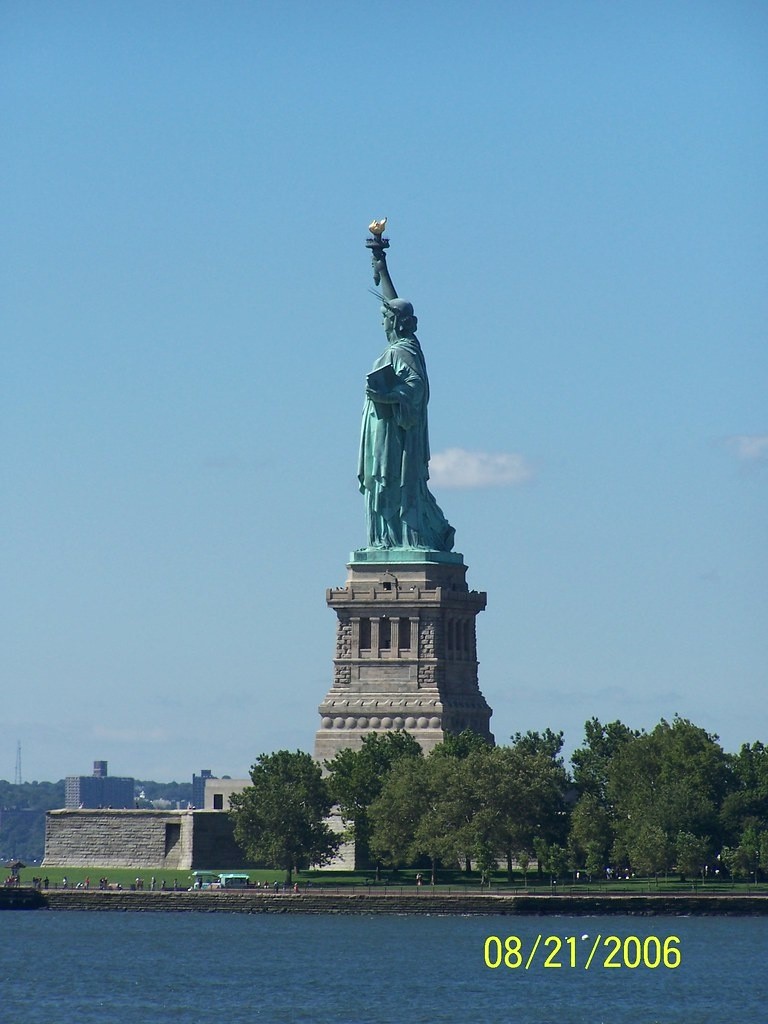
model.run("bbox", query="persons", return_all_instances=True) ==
[351,218,456,553]
[3,873,315,894]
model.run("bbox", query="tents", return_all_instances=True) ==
[190,870,220,891]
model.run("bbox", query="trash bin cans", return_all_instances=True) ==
[129,883,137,891]
